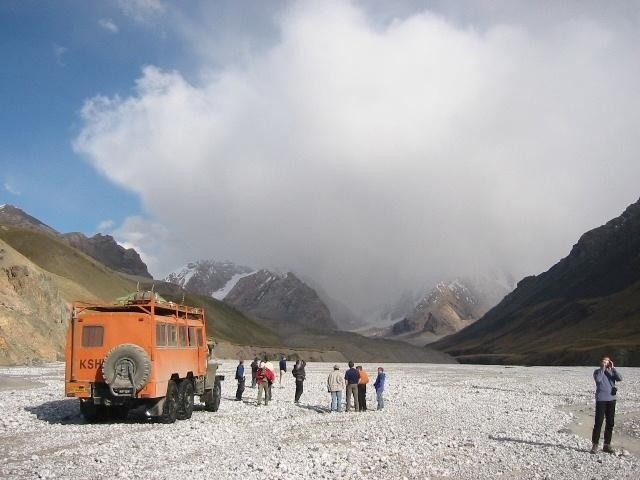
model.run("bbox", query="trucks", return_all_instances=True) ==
[64,281,225,425]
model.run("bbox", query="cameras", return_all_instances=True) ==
[611,387,617,394]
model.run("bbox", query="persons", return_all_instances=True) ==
[327,364,345,412]
[373,367,386,411]
[590,355,623,454]
[291,359,306,403]
[279,355,286,388]
[356,365,369,411]
[344,361,361,412]
[250,357,276,406]
[235,360,245,400]
